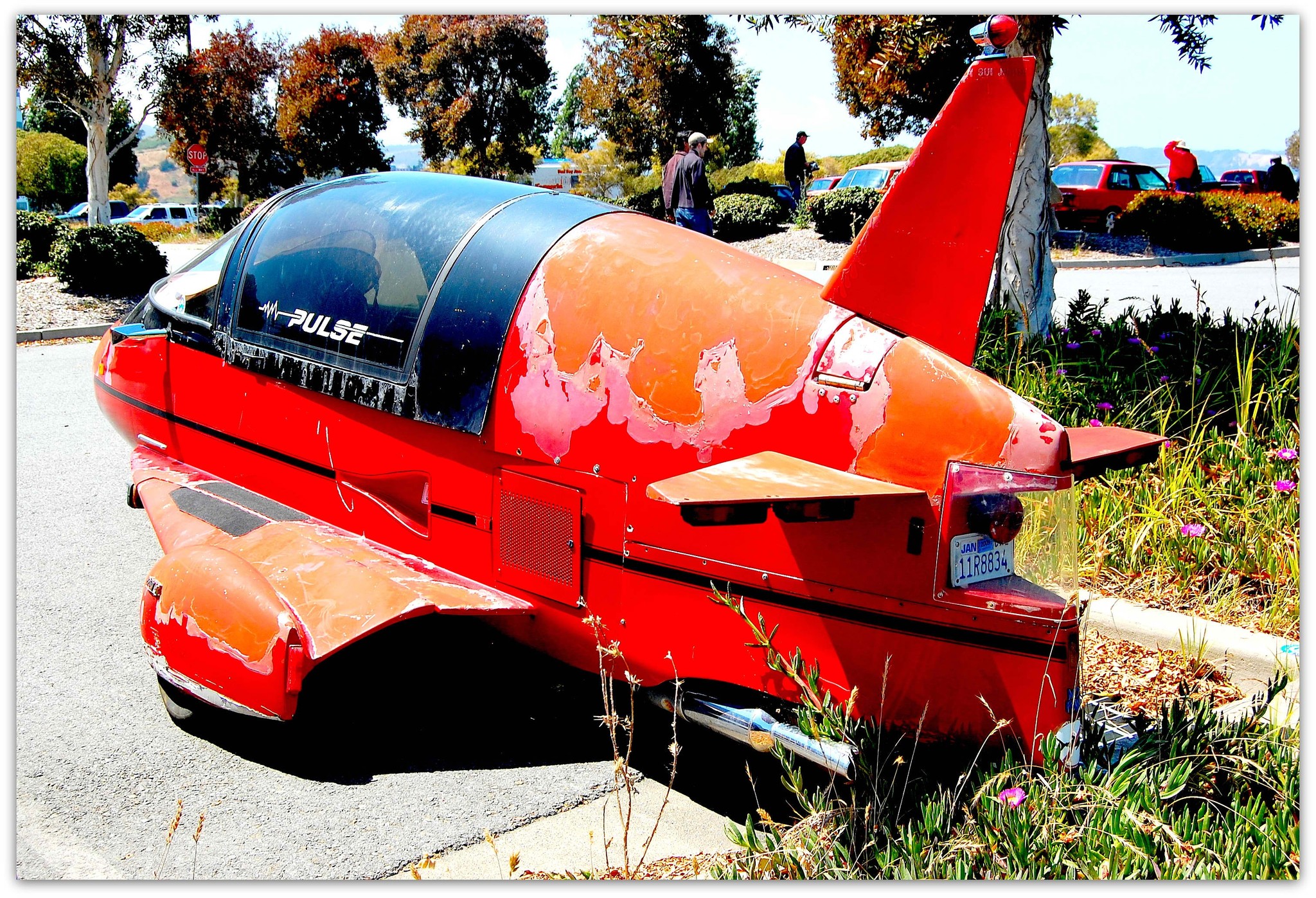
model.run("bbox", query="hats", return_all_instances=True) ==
[688,132,714,145]
[796,131,810,140]
[1175,141,1190,150]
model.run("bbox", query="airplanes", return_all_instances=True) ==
[90,18,1167,805]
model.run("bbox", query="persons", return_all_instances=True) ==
[1264,156,1299,202]
[784,131,810,212]
[662,130,693,225]
[675,132,716,237]
[1164,140,1202,192]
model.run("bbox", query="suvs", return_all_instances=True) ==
[524,159,908,227]
[1045,153,1166,231]
[1141,164,1276,211]
[18,189,225,230]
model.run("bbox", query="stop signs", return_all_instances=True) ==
[184,141,210,168]
